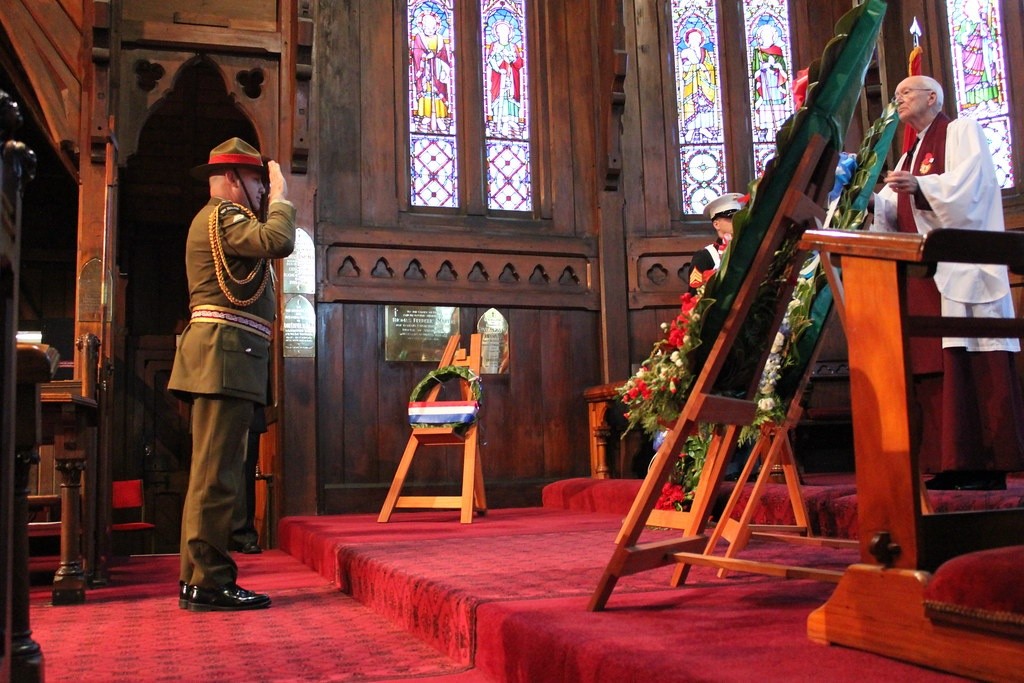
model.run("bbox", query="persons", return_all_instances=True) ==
[867,76,1023,490]
[688,193,759,482]
[166,137,297,612]
[228,404,279,554]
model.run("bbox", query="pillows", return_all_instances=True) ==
[922,543,1024,638]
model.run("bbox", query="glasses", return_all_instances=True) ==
[891,87,936,103]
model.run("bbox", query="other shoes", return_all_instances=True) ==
[924,469,1007,491]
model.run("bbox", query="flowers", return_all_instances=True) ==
[737,311,815,448]
[654,422,716,512]
[615,291,706,441]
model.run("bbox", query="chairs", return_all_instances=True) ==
[113,478,156,555]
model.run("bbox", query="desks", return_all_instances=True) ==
[40,380,112,608]
[796,224,1024,683]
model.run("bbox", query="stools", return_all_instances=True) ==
[582,379,642,480]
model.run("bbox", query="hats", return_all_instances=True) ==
[190,136,268,183]
[703,192,747,221]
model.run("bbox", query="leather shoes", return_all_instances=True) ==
[178,582,270,612]
[241,543,261,554]
